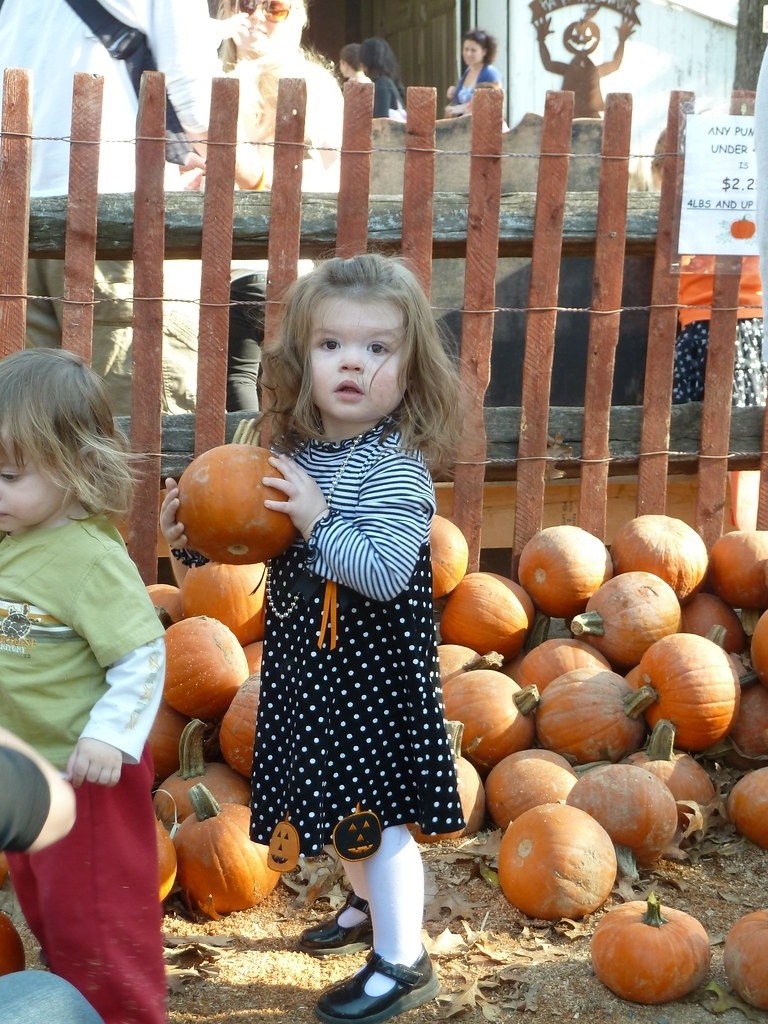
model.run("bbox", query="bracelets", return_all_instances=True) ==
[243,165,266,193]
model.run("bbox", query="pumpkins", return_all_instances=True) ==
[405,513,768,1010]
[0,849,26,976]
[135,417,284,917]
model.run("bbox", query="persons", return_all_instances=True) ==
[0,727,109,1023]
[444,30,508,136]
[207,11,253,51]
[178,52,328,416]
[208,1,344,194]
[651,127,768,410]
[0,347,172,1024]
[360,37,406,118]
[157,251,472,1024]
[2,1,274,418]
[339,44,373,83]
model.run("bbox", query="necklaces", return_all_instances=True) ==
[266,428,380,619]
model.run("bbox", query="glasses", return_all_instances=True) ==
[238,0,292,23]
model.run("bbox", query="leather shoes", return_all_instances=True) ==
[298,891,374,957]
[315,943,440,1024]
